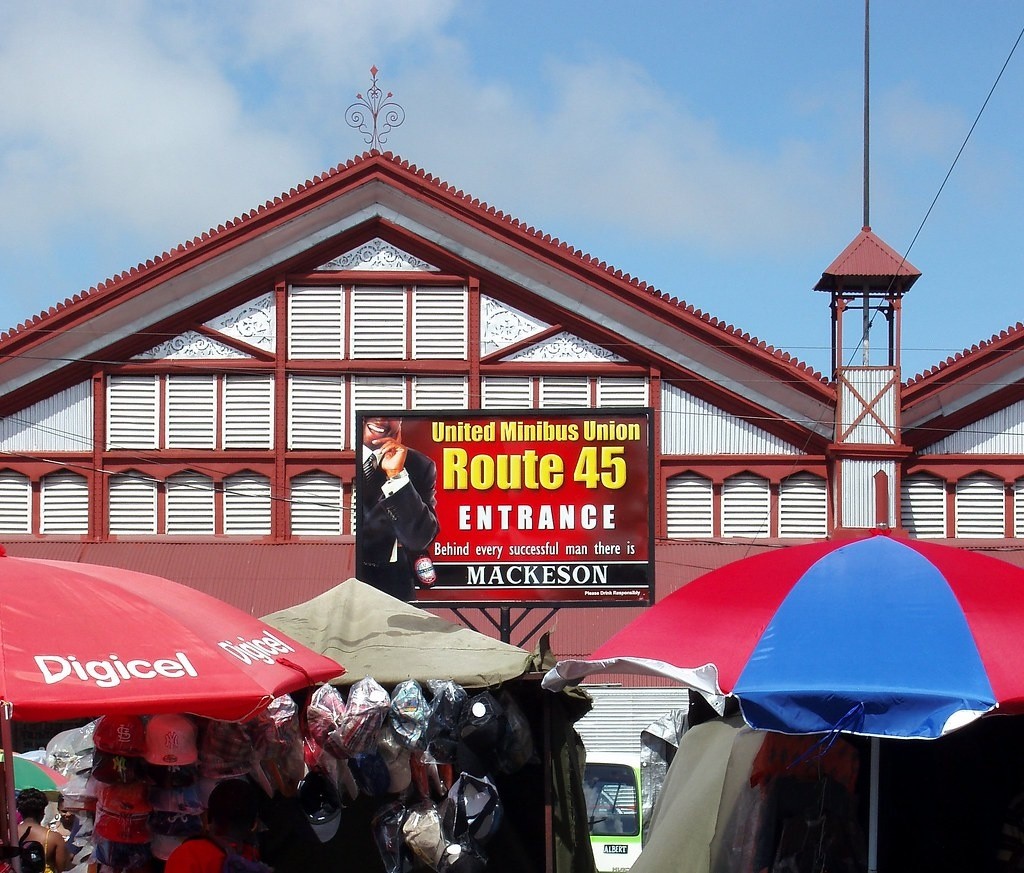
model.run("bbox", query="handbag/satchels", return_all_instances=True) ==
[19,825,46,873]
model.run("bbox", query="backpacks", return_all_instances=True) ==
[189,831,269,873]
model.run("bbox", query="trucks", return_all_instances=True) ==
[572,682,691,872]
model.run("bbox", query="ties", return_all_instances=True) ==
[362,454,377,499]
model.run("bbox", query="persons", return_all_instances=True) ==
[7,788,73,873]
[44,794,72,842]
[165,779,260,873]
[361,416,440,600]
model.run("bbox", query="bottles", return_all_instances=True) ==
[407,549,438,589]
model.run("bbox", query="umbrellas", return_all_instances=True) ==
[0,749,70,790]
[542,522,1024,873]
[0,547,346,873]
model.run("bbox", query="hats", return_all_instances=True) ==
[347,753,390,795]
[377,727,412,794]
[297,770,342,842]
[208,778,260,826]
[460,690,504,738]
[89,680,203,872]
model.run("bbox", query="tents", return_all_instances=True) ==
[257,576,600,873]
